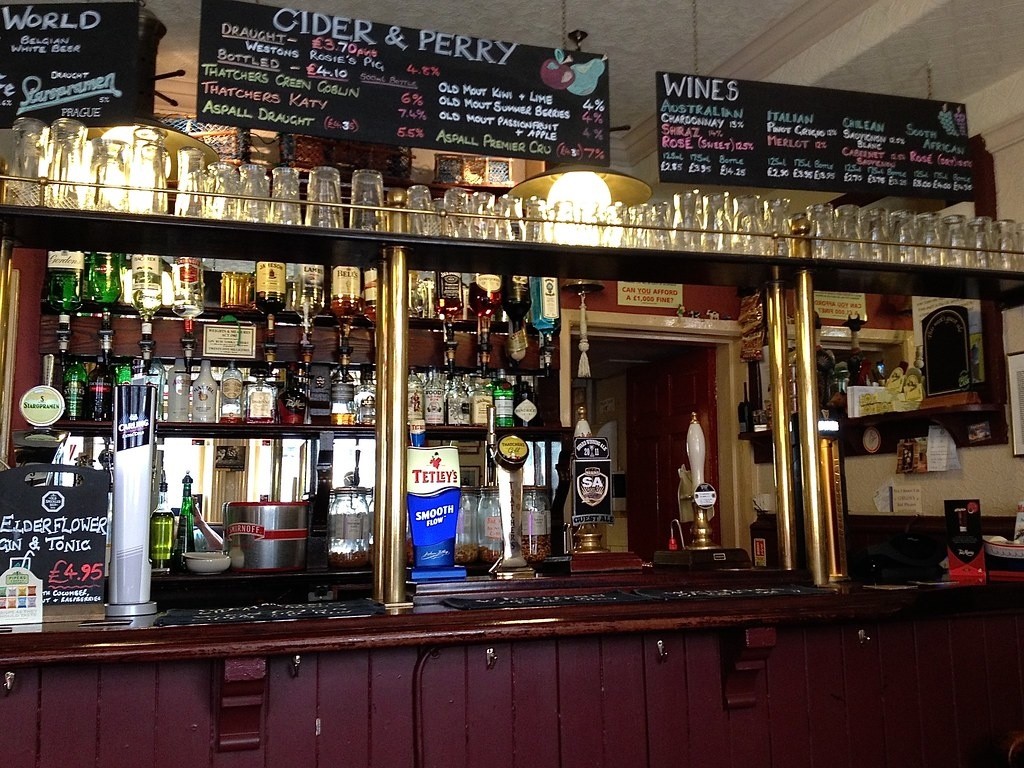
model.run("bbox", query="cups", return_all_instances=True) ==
[0,120,1023,272]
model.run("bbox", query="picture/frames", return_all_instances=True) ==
[461,466,482,487]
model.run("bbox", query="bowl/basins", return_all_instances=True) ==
[185,559,231,575]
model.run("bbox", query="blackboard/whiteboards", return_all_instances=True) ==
[0,464,110,622]
[194,0,611,168]
[653,71,974,197]
[0,2,141,129]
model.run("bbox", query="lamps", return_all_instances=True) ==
[504,0,653,212]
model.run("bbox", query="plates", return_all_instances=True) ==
[180,553,227,560]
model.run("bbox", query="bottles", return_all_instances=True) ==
[30,252,552,570]
